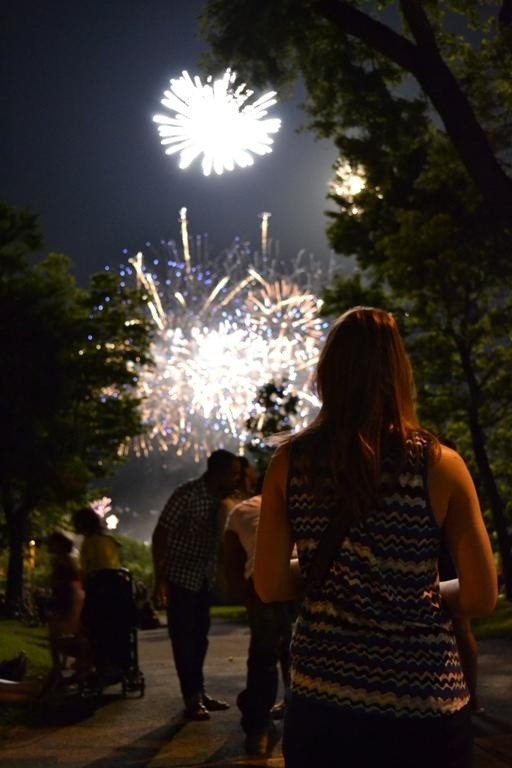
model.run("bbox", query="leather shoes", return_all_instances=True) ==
[185,692,229,720]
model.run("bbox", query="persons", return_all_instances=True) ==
[74,506,123,594]
[37,530,84,678]
[252,307,500,768]
[152,451,302,751]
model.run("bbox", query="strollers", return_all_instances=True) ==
[80,568,160,697]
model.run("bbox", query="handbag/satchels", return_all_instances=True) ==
[136,582,161,630]
